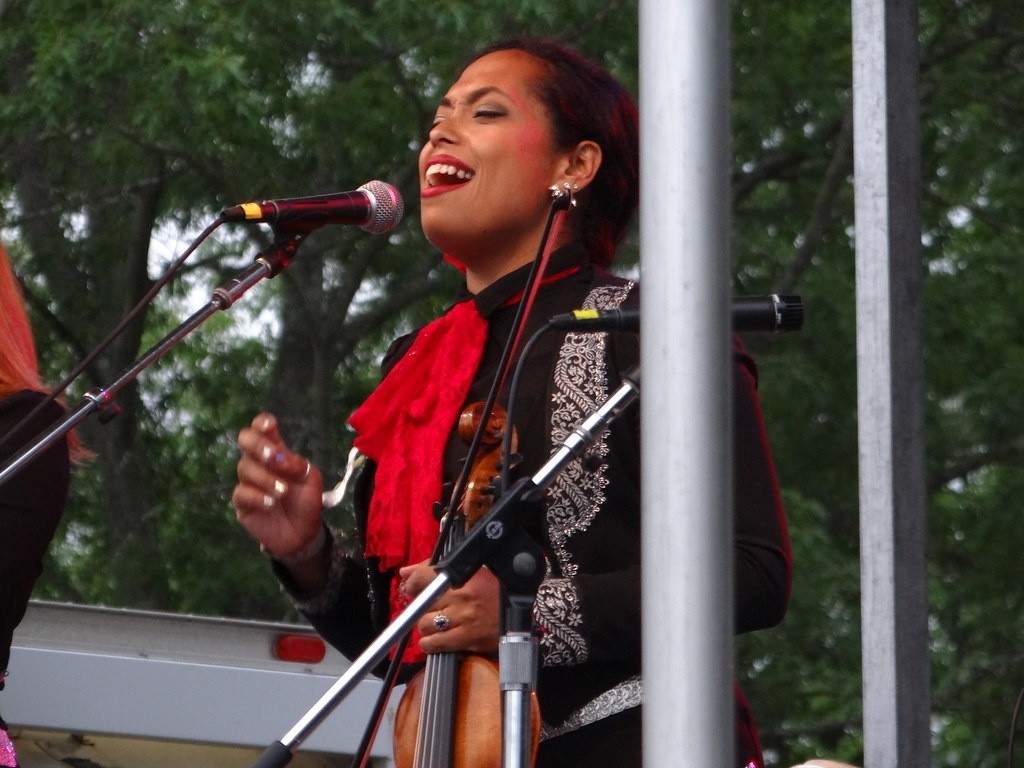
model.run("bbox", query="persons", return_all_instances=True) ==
[230,40,790,767]
[0,252,72,767]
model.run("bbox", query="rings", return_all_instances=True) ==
[303,462,311,480]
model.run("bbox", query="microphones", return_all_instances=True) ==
[547,293,804,334]
[221,180,404,235]
